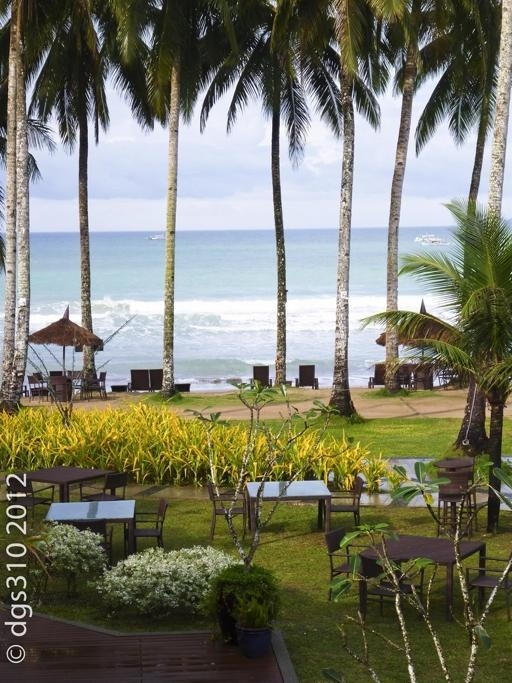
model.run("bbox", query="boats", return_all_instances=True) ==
[414,232,452,246]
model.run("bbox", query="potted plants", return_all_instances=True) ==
[218,562,282,658]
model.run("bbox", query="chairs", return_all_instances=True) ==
[249,363,320,390]
[21,364,191,401]
[368,362,459,390]
